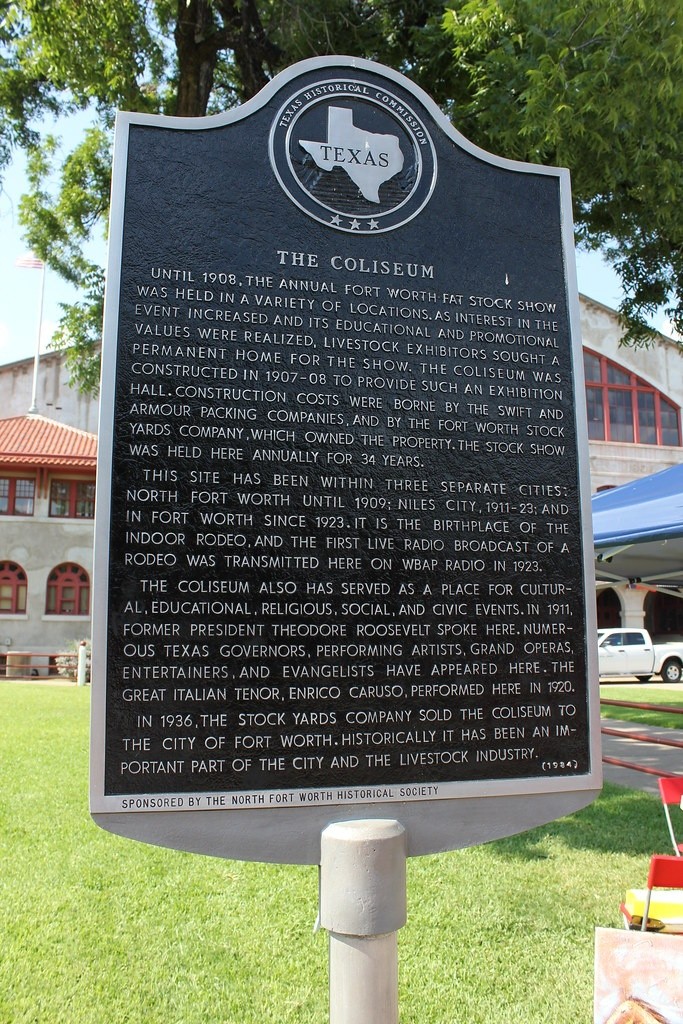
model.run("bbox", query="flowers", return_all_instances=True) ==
[55,637,91,681]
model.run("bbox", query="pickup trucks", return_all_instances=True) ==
[596,627,683,684]
